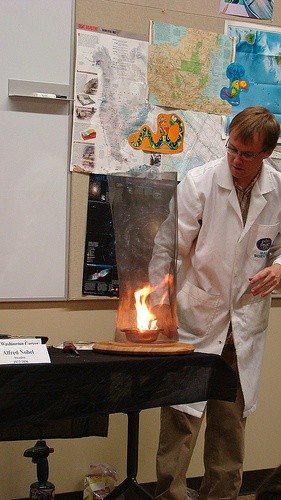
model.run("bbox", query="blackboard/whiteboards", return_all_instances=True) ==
[0,0,75,303]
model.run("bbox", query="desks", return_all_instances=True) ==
[0,341,237,500]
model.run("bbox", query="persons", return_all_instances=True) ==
[147,105,281,500]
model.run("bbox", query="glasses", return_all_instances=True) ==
[225,138,264,160]
